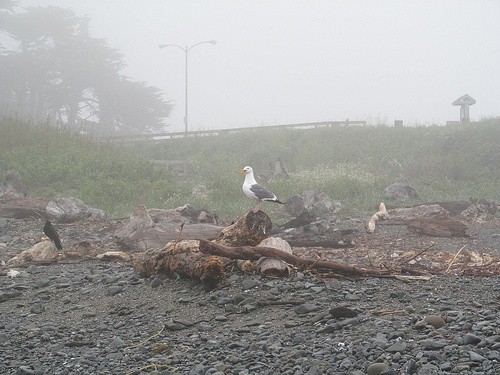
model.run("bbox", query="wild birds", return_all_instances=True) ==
[43,220,63,250]
[327,307,358,321]
[240,166,286,214]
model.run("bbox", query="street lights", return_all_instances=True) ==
[158,40,217,137]
